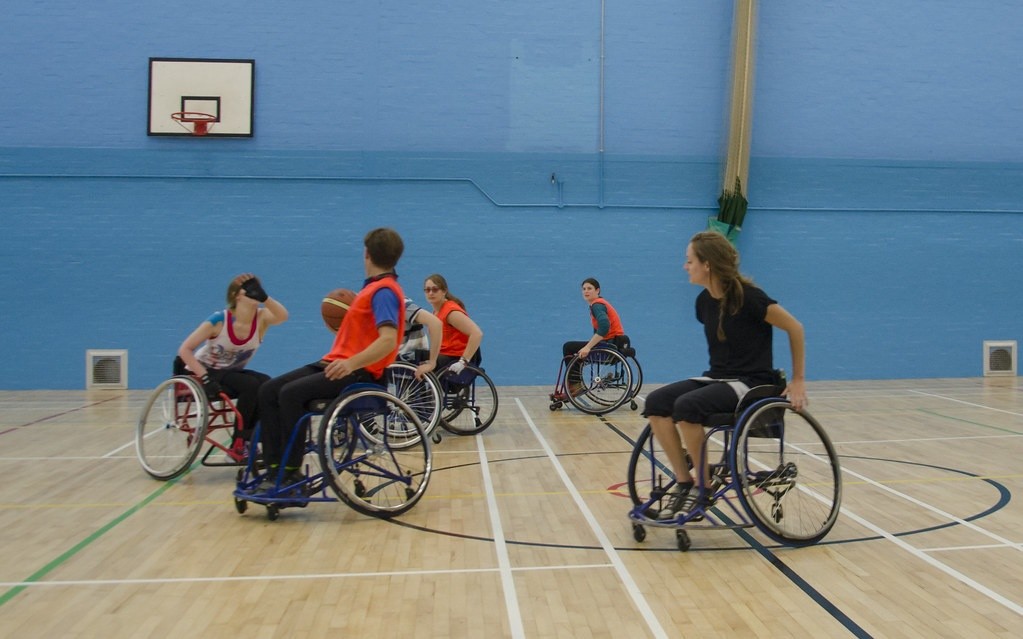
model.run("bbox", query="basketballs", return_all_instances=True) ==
[322,288,358,331]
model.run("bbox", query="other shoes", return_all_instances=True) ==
[232,464,308,507]
[549,383,585,400]
[658,477,715,519]
[225,437,264,464]
[387,409,433,425]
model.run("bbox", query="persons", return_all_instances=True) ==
[553,278,625,399]
[331,269,442,444]
[246,228,405,496]
[176,273,288,465]
[638,232,809,521]
[397,274,483,422]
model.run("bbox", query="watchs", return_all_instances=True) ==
[460,356,468,364]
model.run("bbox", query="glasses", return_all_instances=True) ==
[423,288,442,293]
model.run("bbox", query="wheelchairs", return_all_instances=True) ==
[232,361,499,521]
[549,334,643,415]
[134,355,313,482]
[627,368,844,552]
